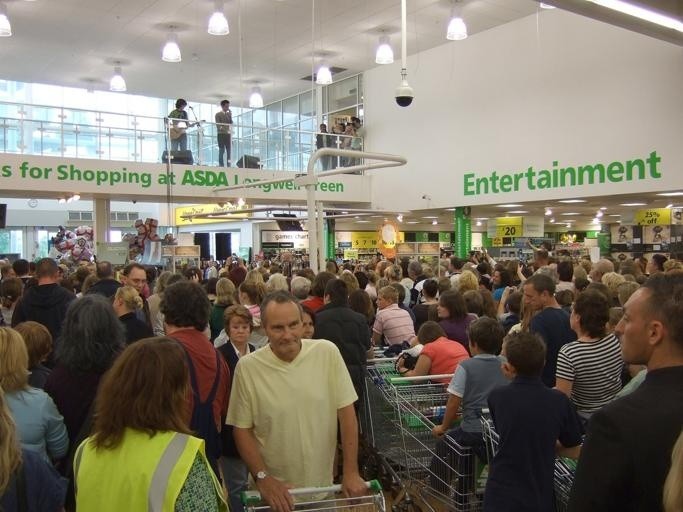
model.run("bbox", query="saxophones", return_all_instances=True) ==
[225,109,232,135]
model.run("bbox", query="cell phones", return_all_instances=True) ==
[232,253,237,263]
[519,248,523,265]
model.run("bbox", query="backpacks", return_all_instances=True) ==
[172,337,223,487]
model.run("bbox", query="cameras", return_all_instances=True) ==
[345,122,355,126]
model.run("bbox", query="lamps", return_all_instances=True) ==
[445,7,469,41]
[375,35,395,65]
[247,85,265,109]
[206,0,231,36]
[108,65,130,93]
[160,32,184,64]
[0,1,16,39]
[315,60,333,85]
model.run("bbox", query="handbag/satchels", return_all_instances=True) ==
[395,352,419,374]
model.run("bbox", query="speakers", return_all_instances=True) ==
[162,150,193,165]
[236,155,260,168]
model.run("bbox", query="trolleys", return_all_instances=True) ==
[356,351,581,512]
[240,478,386,512]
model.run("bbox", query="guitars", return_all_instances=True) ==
[170,120,205,140]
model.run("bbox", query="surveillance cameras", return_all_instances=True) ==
[393,86,414,105]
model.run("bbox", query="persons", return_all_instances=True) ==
[0,238,682,512]
[163,99,198,150]
[316,116,366,174]
[215,99,234,167]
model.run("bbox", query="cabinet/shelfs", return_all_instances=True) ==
[302,247,379,262]
